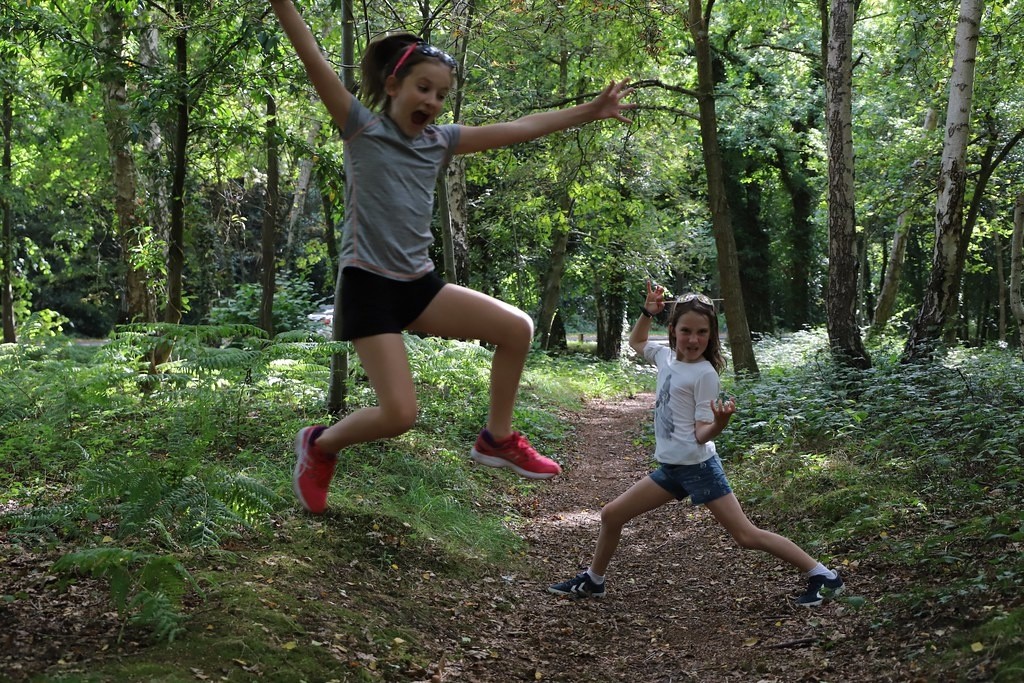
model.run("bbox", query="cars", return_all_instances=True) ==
[307,305,336,327]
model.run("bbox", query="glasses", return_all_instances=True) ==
[672,293,714,313]
[393,42,457,75]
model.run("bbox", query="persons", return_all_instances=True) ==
[270,0,635,512]
[548,279,845,609]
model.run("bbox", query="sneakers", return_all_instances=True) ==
[799,570,846,607]
[294,425,336,513]
[547,573,607,597]
[471,430,562,480]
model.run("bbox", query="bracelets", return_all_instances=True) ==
[639,305,655,317]
